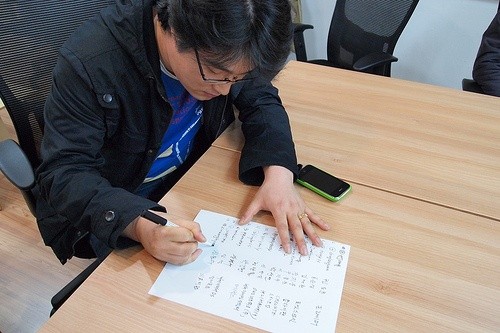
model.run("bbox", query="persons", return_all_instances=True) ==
[34,0,329,265]
[472,10,500,97]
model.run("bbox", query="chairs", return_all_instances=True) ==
[0,0,315,264]
[293,0,419,77]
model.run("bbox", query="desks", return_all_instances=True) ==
[31,59,500,333]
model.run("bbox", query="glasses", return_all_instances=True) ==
[193,47,260,84]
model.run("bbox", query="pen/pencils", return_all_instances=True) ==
[140,208,215,248]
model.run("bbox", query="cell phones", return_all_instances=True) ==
[296,164,352,202]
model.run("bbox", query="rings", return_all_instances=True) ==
[298,213,308,220]
